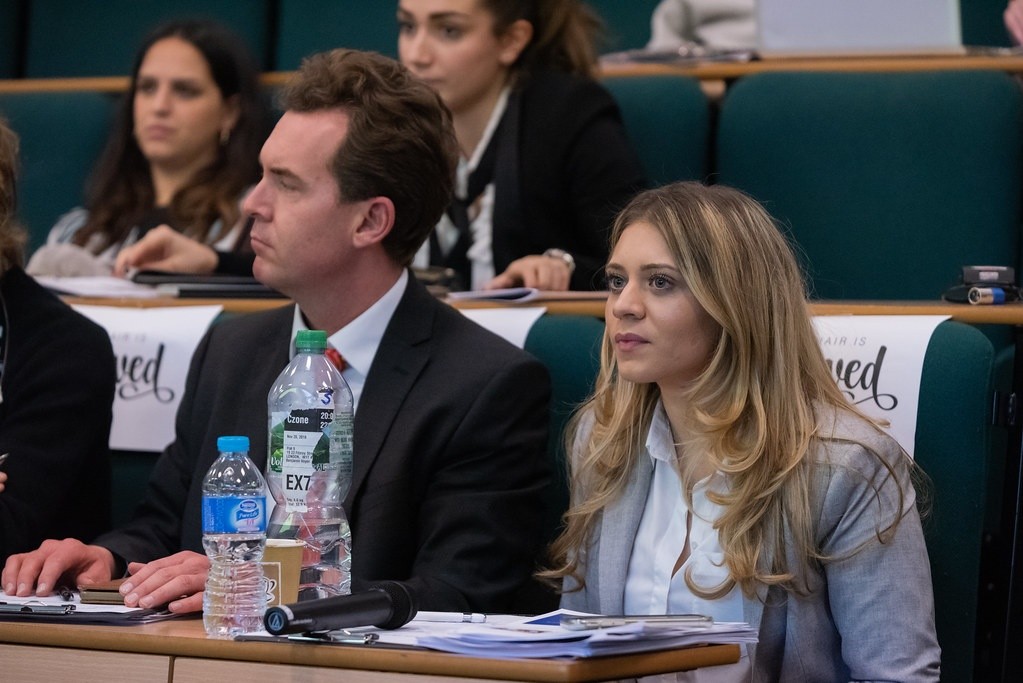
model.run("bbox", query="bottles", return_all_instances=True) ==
[201,436,271,639]
[268,328,352,599]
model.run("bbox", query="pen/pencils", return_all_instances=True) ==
[413,611,486,623]
[58,582,75,601]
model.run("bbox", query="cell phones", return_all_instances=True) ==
[80,588,124,605]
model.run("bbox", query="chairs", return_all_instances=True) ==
[71,302,225,536]
[714,68,1022,304]
[600,69,709,192]
[24,0,272,82]
[459,307,613,530]
[585,0,660,58]
[279,2,399,73]
[0,88,125,263]
[805,316,996,683]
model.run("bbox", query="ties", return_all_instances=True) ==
[427,112,500,291]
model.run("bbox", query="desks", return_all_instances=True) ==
[1,609,741,683]
[208,291,1023,327]
[2,46,1023,96]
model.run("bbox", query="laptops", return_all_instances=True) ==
[753,0,1019,58]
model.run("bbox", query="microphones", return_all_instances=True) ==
[263,581,419,635]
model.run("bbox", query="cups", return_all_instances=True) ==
[262,538,306,608]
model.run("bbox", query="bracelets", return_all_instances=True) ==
[541,245,575,275]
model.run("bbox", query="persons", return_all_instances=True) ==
[559,181,945,683]
[646,2,758,100]
[0,50,558,621]
[1003,0,1019,47]
[0,119,118,580]
[43,20,281,296]
[110,1,632,291]
